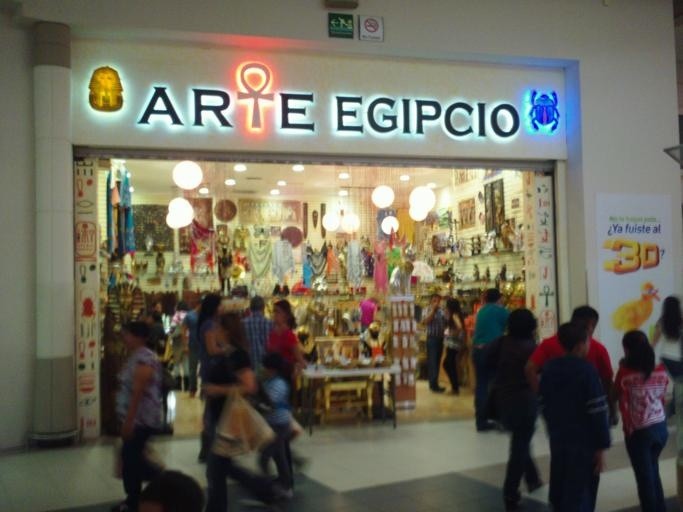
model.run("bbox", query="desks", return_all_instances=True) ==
[298,366,403,436]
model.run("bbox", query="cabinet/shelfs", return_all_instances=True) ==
[449,247,525,286]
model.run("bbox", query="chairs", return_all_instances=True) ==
[308,336,375,422]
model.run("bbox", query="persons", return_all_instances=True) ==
[651,295,682,418]
[419,287,510,435]
[613,328,671,511]
[523,303,619,434]
[108,286,303,511]
[473,307,543,509]
[537,320,613,512]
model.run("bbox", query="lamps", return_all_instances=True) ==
[162,160,452,235]
